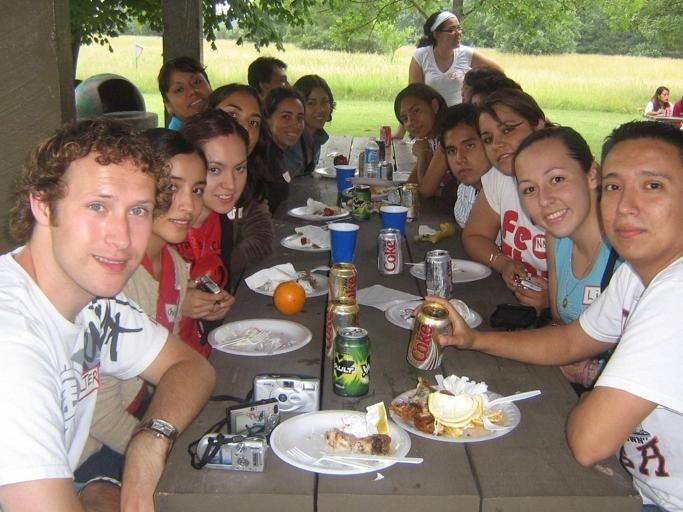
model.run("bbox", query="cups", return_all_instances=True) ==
[380,205,407,244]
[336,165,356,194]
[329,224,360,265]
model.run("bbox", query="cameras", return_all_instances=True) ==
[254,374,322,413]
[194,430,267,475]
[227,398,280,443]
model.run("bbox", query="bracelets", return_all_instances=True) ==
[576,358,605,388]
[487,251,504,270]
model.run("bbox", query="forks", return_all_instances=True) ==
[481,416,509,432]
[287,446,368,472]
[210,330,270,349]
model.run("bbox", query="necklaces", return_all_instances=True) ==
[562,235,602,308]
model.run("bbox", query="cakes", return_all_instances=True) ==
[394,374,502,438]
[325,402,391,457]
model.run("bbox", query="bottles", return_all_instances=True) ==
[380,161,392,181]
[365,136,379,179]
[359,150,366,179]
[377,142,386,164]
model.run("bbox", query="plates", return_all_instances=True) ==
[286,206,349,221]
[313,168,360,179]
[344,200,392,213]
[345,184,393,197]
[268,409,411,476]
[411,258,491,284]
[385,297,485,331]
[388,388,523,445]
[280,230,332,252]
[252,272,328,302]
[209,318,312,357]
[392,172,412,185]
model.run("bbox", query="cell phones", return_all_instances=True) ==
[196,275,224,296]
[515,277,545,294]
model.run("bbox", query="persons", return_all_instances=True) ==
[211,84,274,291]
[394,83,453,197]
[437,103,492,229]
[461,87,557,311]
[284,74,335,177]
[169,108,250,358]
[243,57,289,105]
[0,119,218,511]
[643,86,672,119]
[468,74,522,106]
[512,126,623,390]
[247,88,304,209]
[672,97,683,128]
[461,66,503,103]
[76,128,210,494]
[158,57,214,131]
[391,11,505,186]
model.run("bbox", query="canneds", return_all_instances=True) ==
[378,162,393,182]
[334,327,370,396]
[378,229,403,276]
[330,262,358,303]
[325,297,360,359]
[375,140,385,162]
[405,301,450,370]
[352,186,370,220]
[401,183,419,220]
[359,152,364,177]
[426,250,454,301]
[380,126,391,148]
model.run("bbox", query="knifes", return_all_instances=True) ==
[321,448,423,466]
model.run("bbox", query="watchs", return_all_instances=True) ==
[129,419,179,461]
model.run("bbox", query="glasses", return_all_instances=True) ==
[434,26,462,34]
